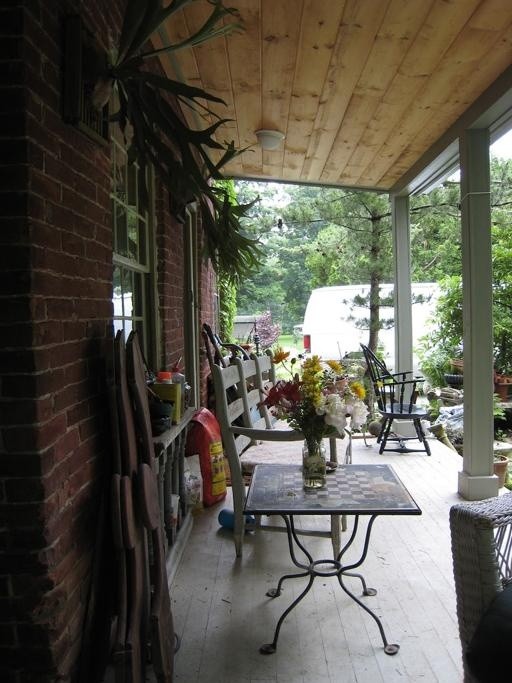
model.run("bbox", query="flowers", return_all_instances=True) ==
[254,347,373,485]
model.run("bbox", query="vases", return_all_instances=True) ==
[302,438,327,488]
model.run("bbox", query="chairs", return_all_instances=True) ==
[446,488,511,682]
[360,343,431,456]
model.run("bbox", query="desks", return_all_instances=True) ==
[242,462,422,655]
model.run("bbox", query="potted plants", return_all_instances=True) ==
[493,392,510,489]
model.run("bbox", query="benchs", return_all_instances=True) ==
[209,349,352,565]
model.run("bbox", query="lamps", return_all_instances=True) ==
[255,129,285,151]
